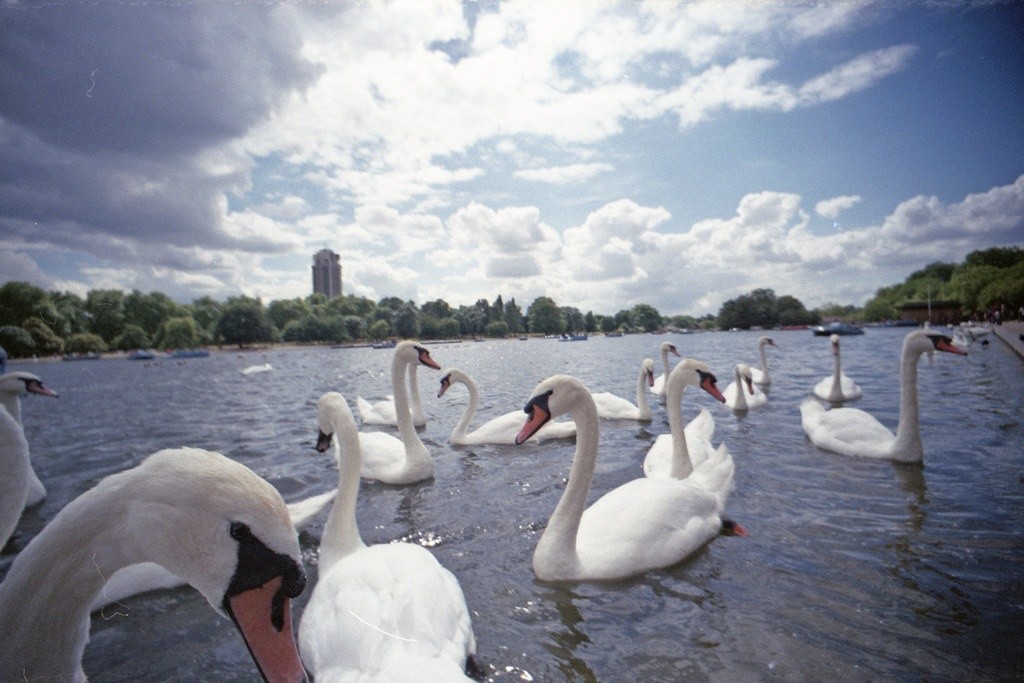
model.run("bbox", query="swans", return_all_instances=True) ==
[0,320,995,683]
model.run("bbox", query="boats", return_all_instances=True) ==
[62,322,743,362]
[811,321,864,336]
